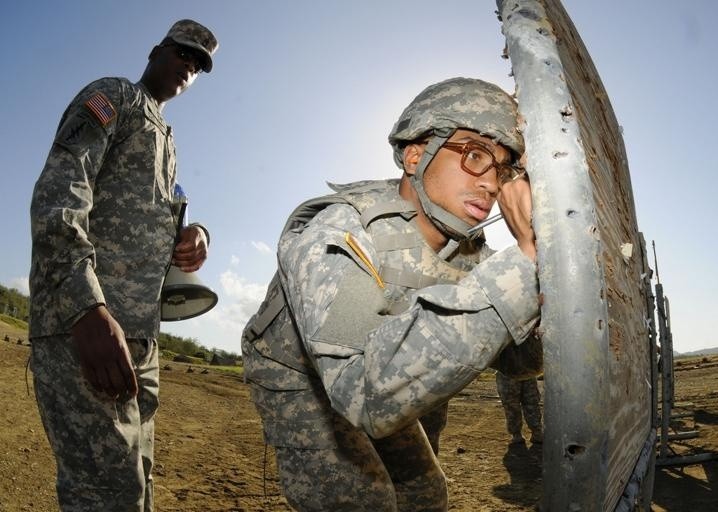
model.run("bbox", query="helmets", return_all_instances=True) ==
[389,77,525,160]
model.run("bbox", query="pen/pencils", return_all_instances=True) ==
[467,212,503,235]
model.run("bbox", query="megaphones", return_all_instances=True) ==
[160,182,218,321]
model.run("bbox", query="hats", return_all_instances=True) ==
[166,20,218,73]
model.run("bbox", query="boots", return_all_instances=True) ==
[530,428,544,444]
[509,433,525,446]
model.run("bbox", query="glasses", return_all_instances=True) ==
[159,43,203,73]
[421,140,525,190]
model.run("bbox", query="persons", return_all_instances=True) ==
[241,78,541,512]
[419,401,448,456]
[28,19,219,511]
[495,372,542,450]
